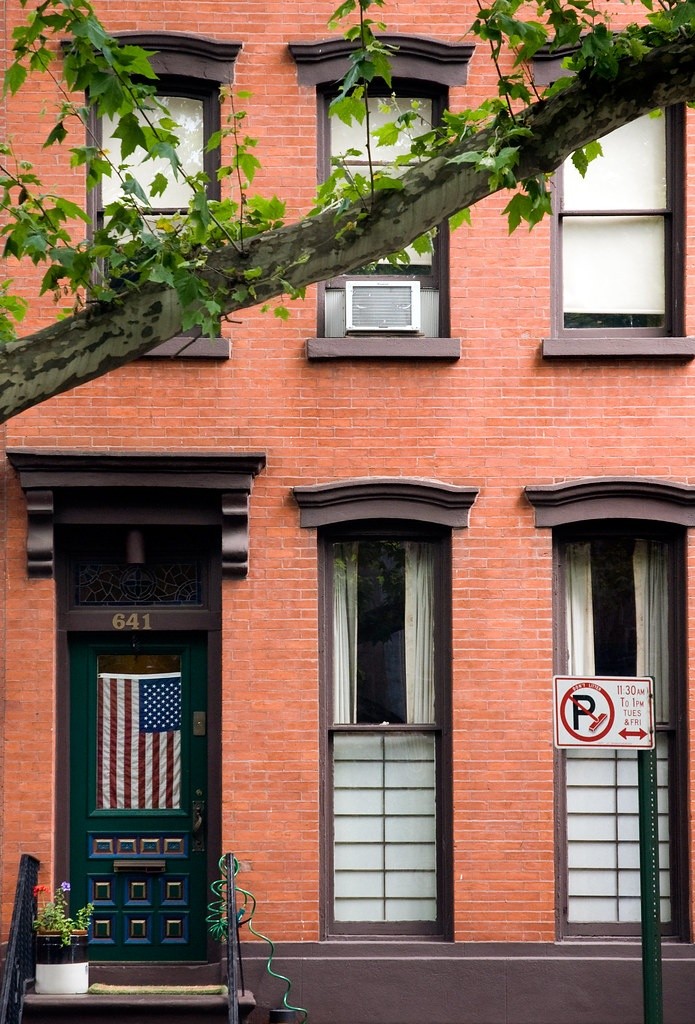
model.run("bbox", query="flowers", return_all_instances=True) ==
[32,881,95,946]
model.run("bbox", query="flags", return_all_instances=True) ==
[96,672,183,810]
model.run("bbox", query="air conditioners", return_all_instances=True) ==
[345,280,421,334]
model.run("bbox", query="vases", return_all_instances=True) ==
[35,930,91,995]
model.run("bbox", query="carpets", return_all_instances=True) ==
[89,983,228,996]
